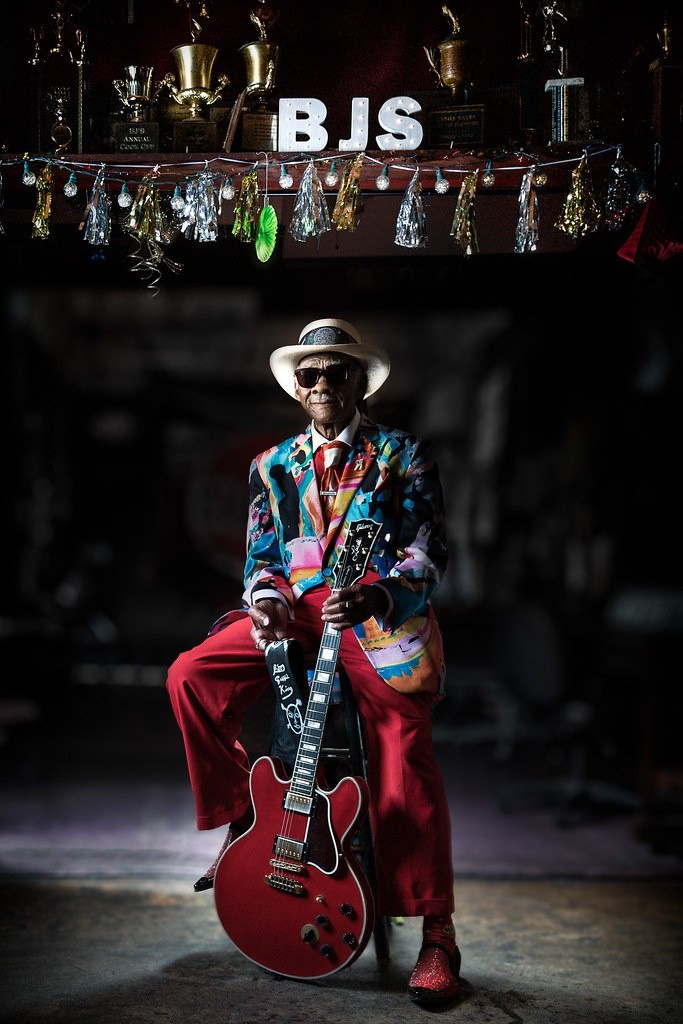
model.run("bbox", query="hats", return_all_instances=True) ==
[269,318,391,402]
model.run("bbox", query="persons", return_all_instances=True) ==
[168,318,464,1004]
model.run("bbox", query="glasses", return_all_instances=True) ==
[295,365,350,388]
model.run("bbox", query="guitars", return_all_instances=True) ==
[210,516,386,983]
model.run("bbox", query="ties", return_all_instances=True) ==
[319,441,345,533]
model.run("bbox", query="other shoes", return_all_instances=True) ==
[408,942,461,1002]
[193,827,245,892]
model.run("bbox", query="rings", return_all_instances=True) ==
[346,601,353,610]
[254,638,263,650]
